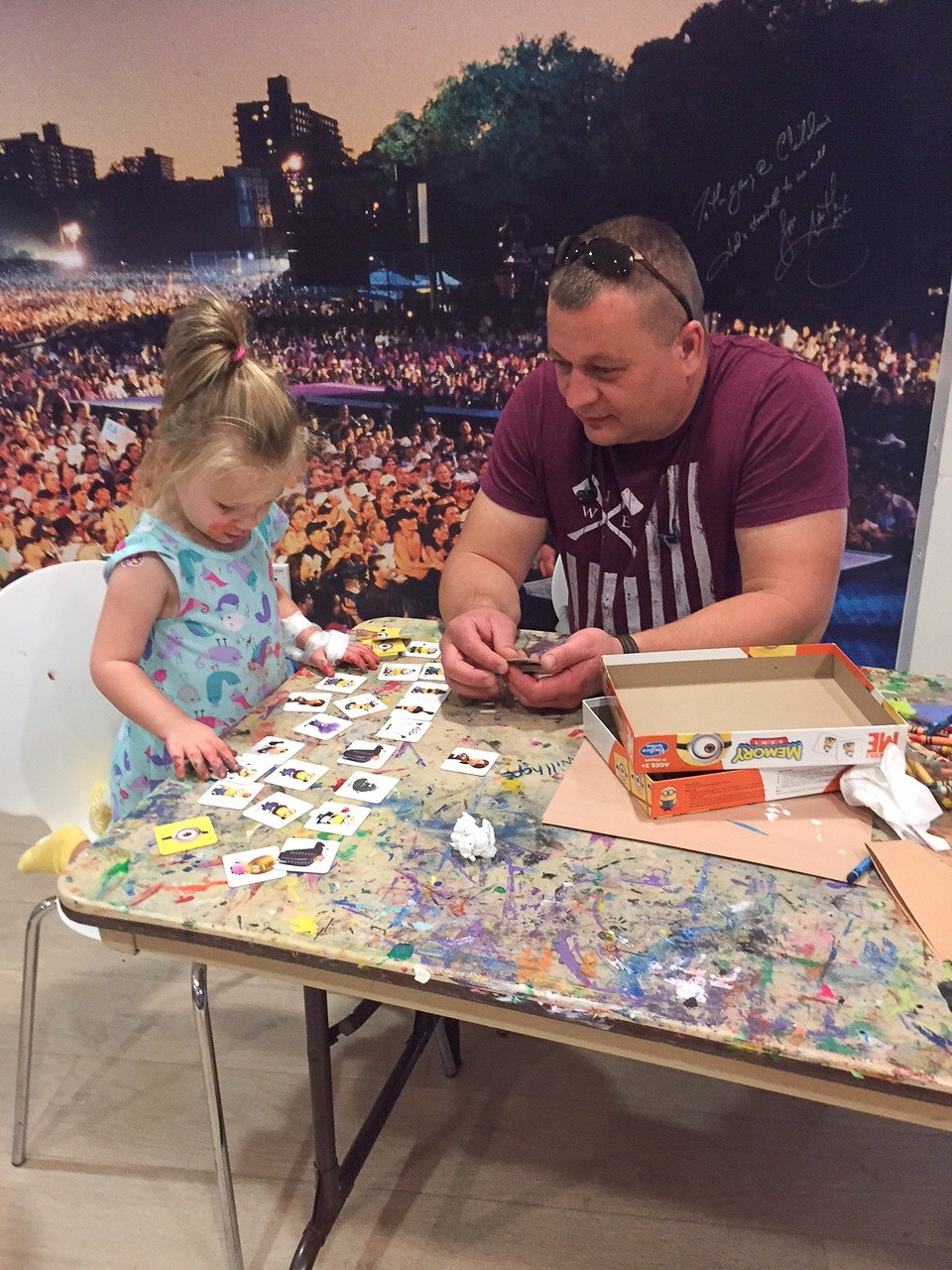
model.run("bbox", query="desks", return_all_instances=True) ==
[52,611,952,1270]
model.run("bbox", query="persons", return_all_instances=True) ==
[16,290,380,877]
[438,217,851,711]
[0,272,942,630]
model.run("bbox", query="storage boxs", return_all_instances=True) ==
[577,639,913,822]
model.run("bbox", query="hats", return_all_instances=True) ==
[115,473,129,485]
[88,480,106,501]
[53,516,79,537]
[288,414,473,573]
[37,488,55,499]
[16,535,36,550]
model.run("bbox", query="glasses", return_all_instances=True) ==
[550,236,695,324]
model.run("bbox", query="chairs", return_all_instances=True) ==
[0,557,460,1270]
